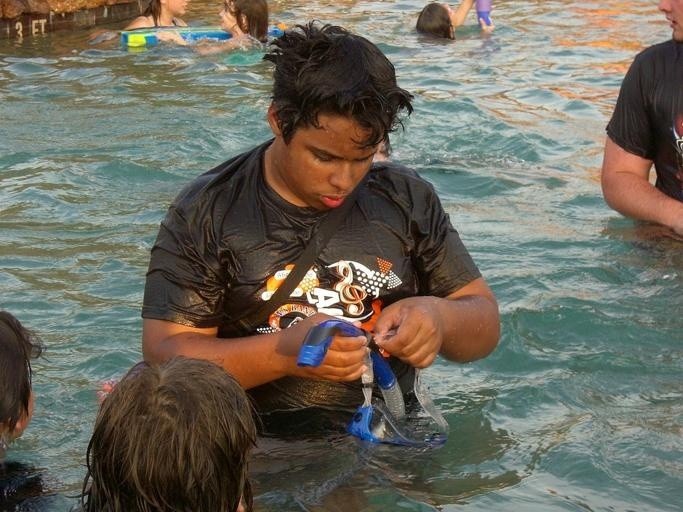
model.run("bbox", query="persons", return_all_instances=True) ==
[90,356,258,512]
[600,0,683,243]
[0,310,44,512]
[70,0,268,55]
[141,24,502,439]
[417,0,496,41]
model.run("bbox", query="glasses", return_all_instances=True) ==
[350,404,448,448]
